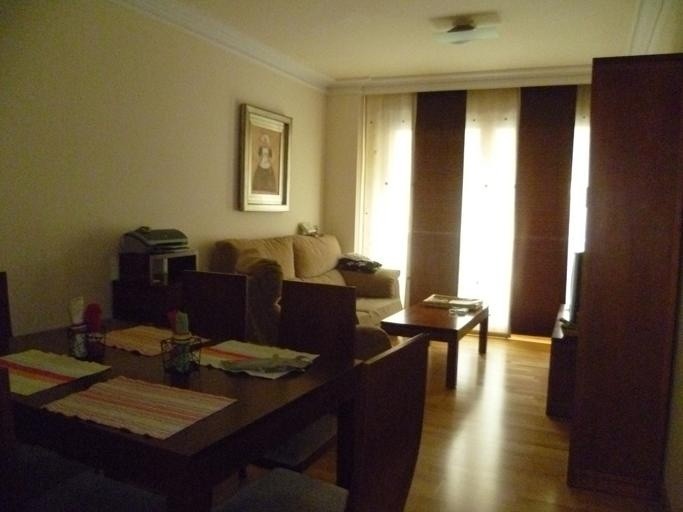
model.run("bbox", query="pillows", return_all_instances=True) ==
[212,233,342,279]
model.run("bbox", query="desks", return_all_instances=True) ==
[546,304,578,418]
[4,317,364,512]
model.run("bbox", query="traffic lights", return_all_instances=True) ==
[212,233,342,279]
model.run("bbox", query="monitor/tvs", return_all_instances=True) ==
[421,293,482,312]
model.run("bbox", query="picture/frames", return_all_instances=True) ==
[546,304,578,418]
[239,101,293,212]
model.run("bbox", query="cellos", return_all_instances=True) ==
[124,226,193,253]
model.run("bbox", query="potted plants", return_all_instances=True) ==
[239,101,293,212]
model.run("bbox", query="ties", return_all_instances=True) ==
[568,252,583,323]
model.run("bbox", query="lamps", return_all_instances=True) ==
[431,11,502,44]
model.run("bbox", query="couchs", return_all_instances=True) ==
[211,233,403,360]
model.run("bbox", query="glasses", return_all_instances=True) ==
[431,11,502,44]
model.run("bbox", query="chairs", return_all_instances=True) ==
[207,333,427,512]
[182,271,250,342]
[237,279,358,484]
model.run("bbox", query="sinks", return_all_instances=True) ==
[211,233,403,360]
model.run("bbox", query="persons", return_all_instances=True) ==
[251,134,277,192]
